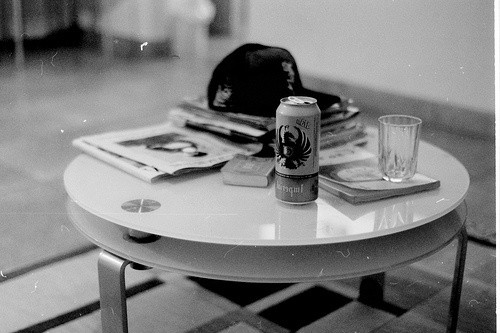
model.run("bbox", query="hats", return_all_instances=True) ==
[206,42,342,118]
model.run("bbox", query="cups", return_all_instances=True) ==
[378,114,423,184]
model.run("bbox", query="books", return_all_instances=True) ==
[71,96,439,203]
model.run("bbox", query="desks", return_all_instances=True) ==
[61,124,473,333]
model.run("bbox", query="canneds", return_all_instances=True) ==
[275,95,321,204]
